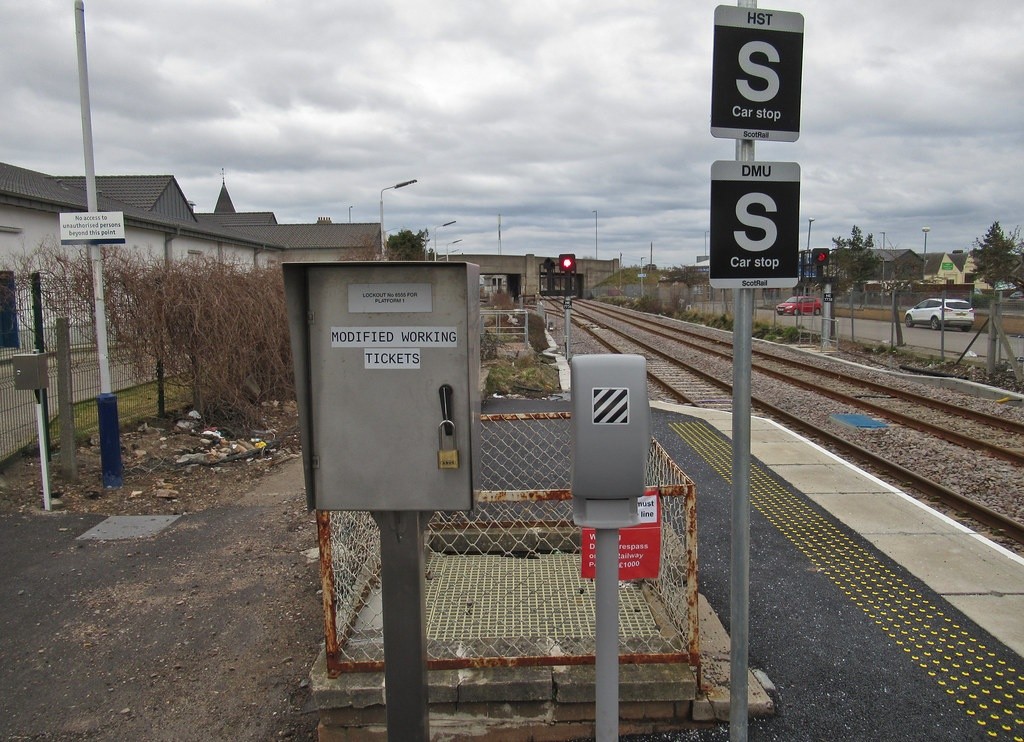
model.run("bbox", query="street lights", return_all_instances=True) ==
[923,228,931,280]
[813,247,830,267]
[803,217,816,302]
[380,179,418,260]
[423,220,463,261]
[593,210,598,260]
[879,231,885,308]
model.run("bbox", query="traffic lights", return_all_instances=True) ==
[558,254,577,271]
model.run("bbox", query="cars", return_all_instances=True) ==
[905,297,975,334]
[776,296,822,316]
